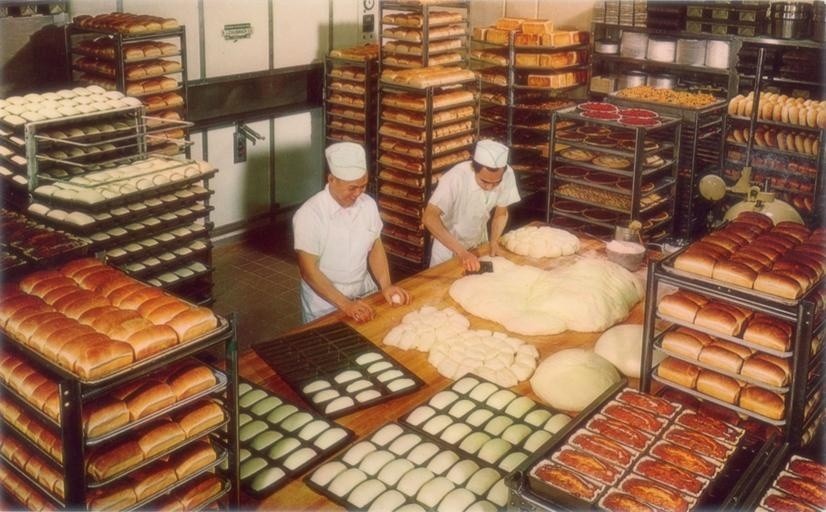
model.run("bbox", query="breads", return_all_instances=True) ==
[70,12,184,156]
[1,334,224,512]
[722,90,826,213]
[649,276,825,448]
[469,18,592,194]
[0,207,86,269]
[672,209,826,299]
[529,386,744,511]
[328,11,476,263]
[550,102,668,243]
[0,258,217,378]
[755,454,826,512]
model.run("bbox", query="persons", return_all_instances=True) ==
[293,165,414,326]
[422,140,521,272]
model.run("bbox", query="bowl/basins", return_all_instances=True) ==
[608,246,646,272]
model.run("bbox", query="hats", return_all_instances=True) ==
[324,142,368,181]
[474,138,509,168]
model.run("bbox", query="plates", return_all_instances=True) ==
[647,37,674,64]
[530,388,745,511]
[595,42,617,54]
[675,38,705,69]
[620,31,647,59]
[647,72,673,89]
[752,455,826,511]
[553,100,667,240]
[706,40,728,68]
[618,70,644,90]
